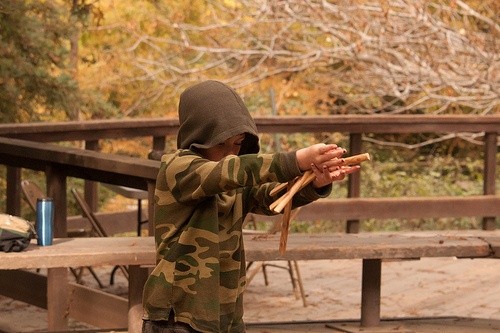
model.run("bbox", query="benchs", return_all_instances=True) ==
[0,227,500,332]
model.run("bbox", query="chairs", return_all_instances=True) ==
[19,180,141,288]
[240,205,308,307]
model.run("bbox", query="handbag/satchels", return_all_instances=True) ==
[0,214,37,252]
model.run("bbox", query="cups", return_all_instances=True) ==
[37,197,56,246]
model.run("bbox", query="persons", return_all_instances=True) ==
[139,78,362,333]
